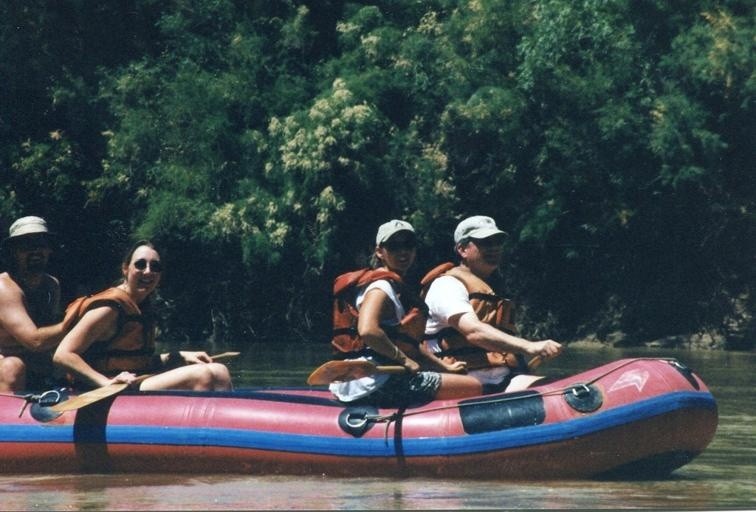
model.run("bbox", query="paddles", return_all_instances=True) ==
[50,350,242,412]
[306,360,408,387]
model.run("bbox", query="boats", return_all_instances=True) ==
[0,356,718,478]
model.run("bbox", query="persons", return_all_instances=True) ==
[54,238,237,394]
[1,216,86,392]
[419,214,563,389]
[327,218,482,403]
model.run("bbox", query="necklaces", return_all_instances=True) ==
[122,282,127,292]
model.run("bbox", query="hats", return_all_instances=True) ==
[375,220,414,246]
[3,215,56,240]
[453,215,508,244]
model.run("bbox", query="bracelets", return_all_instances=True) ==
[391,344,403,362]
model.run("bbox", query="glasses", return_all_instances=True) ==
[383,240,416,250]
[134,260,162,272]
